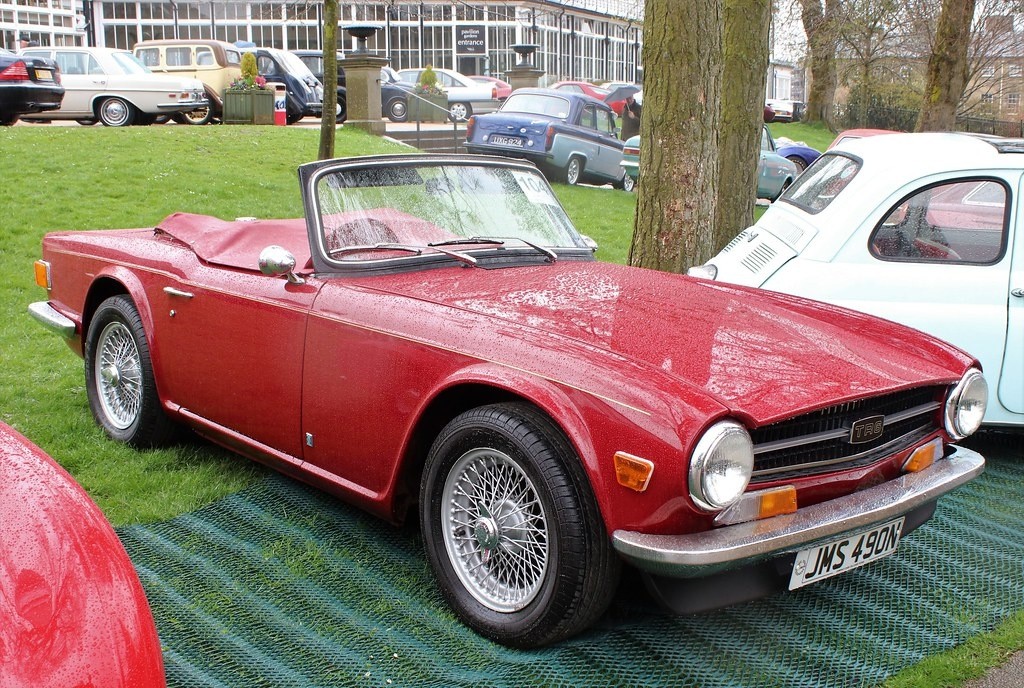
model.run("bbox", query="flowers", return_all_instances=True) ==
[410,63,447,95]
[224,51,276,91]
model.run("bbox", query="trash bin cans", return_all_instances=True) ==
[266,82,287,126]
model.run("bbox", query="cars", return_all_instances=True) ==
[25,148,986,651]
[681,134,1024,429]
[396,68,500,123]
[600,79,642,106]
[816,129,1009,234]
[762,103,776,123]
[290,47,347,124]
[133,39,245,126]
[619,124,797,204]
[772,135,820,174]
[196,40,324,125]
[0,46,65,126]
[17,46,209,127]
[765,97,793,123]
[462,87,635,193]
[0,416,168,688]
[466,76,512,102]
[785,98,804,121]
[380,65,417,123]
[547,81,627,118]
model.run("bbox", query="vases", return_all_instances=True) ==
[223,89,275,125]
[407,94,448,122]
